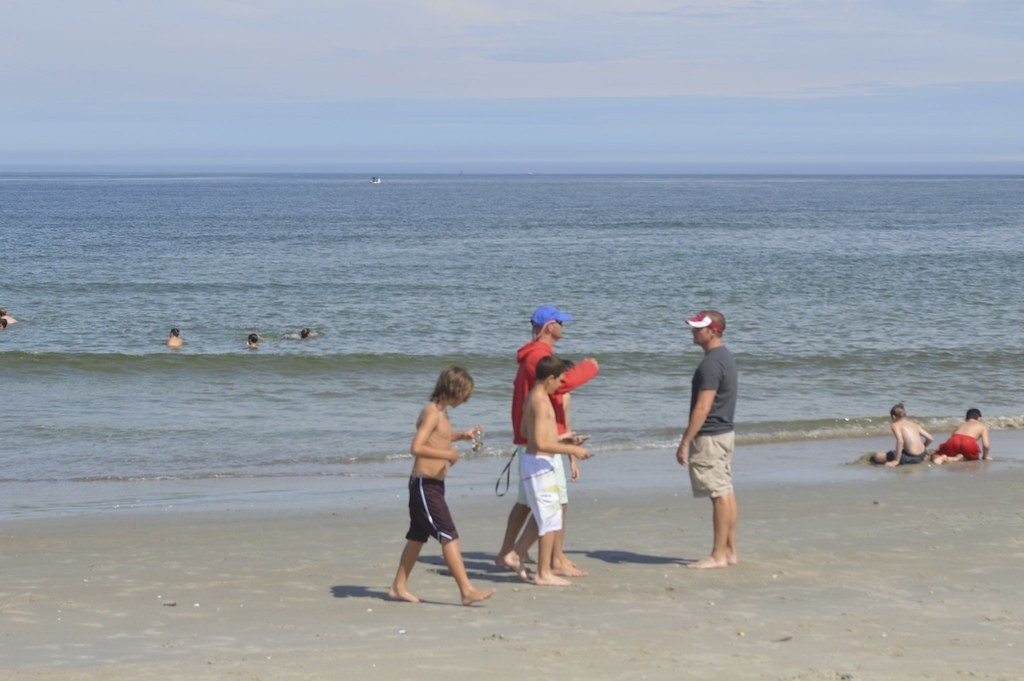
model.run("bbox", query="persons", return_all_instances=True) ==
[495,305,600,586]
[283,327,318,341]
[676,310,738,570]
[387,364,494,606]
[166,328,184,347]
[244,334,262,349]
[930,408,994,465]
[870,403,933,468]
[0,308,19,330]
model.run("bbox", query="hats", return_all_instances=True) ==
[686,313,724,333]
[531,305,572,327]
